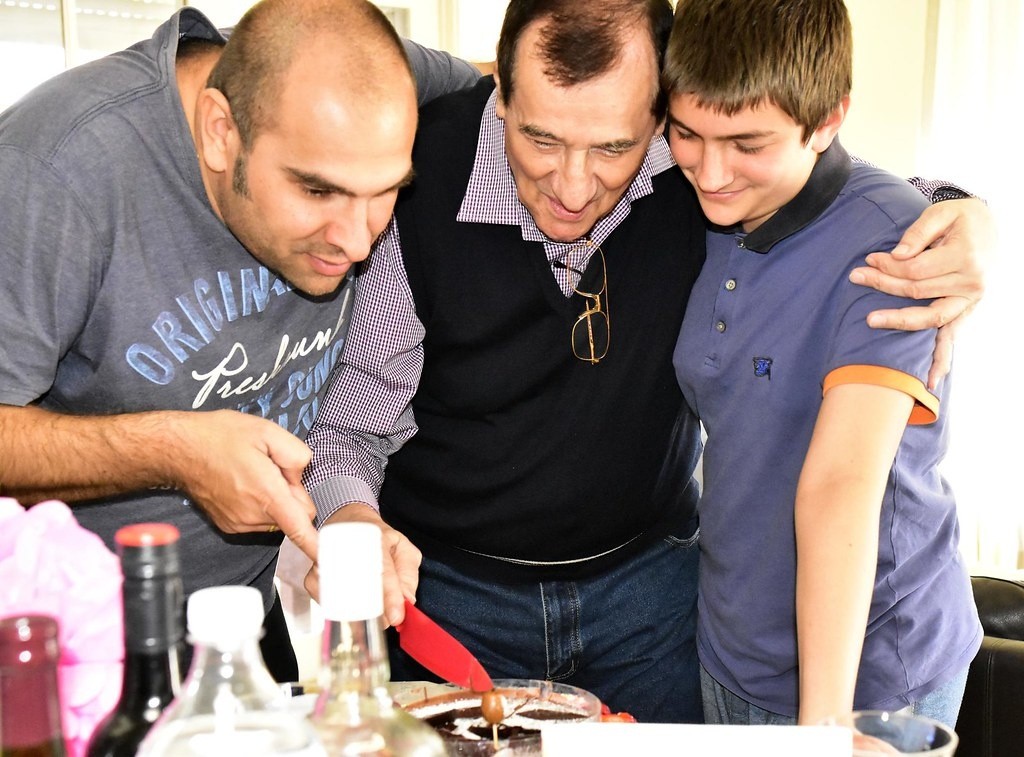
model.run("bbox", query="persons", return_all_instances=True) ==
[302,0,996,728]
[659,0,987,757]
[0,0,480,757]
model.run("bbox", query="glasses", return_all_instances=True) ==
[554,242,610,365]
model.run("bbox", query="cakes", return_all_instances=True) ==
[396,685,598,757]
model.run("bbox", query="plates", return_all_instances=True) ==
[395,678,603,757]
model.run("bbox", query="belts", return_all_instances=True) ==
[415,479,698,586]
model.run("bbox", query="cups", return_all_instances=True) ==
[817,710,959,757]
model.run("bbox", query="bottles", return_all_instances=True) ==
[302,520,451,757]
[84,523,194,757]
[135,582,328,757]
[0,614,68,757]
